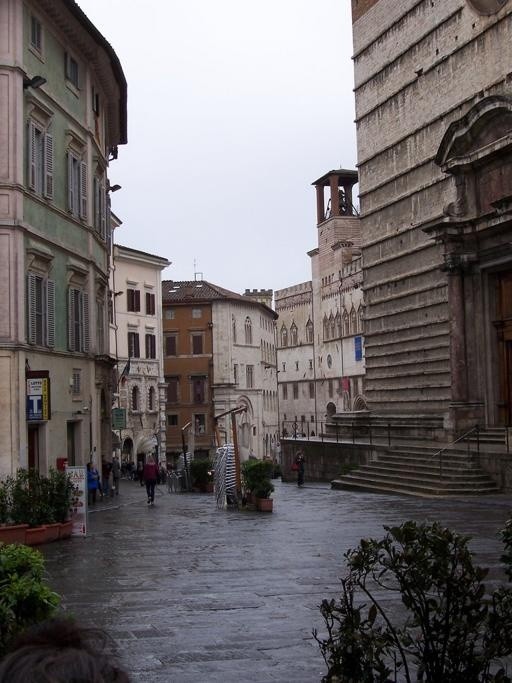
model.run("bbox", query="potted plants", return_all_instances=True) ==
[256,479,275,512]
[60,470,73,538]
[0,468,29,545]
[26,468,47,544]
[42,464,61,541]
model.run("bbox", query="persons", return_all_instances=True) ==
[294,448,305,487]
[86,463,99,506]
[142,457,160,505]
[101,455,144,497]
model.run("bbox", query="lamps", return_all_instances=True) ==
[108,185,121,193]
[23,75,47,90]
